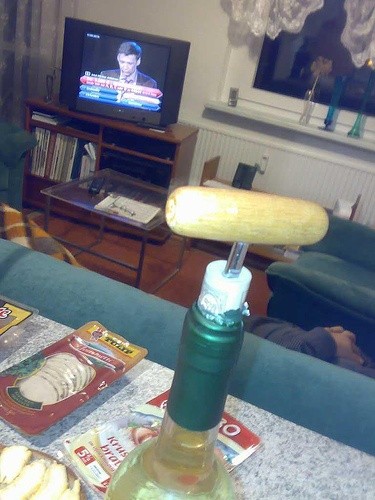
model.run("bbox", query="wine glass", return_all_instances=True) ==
[318,74,347,131]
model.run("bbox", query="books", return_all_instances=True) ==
[66,388,262,500]
[30,111,158,223]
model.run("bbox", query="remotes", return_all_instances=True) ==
[88,176,105,195]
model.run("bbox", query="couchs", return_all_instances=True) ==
[0,214,375,457]
[0,120,36,212]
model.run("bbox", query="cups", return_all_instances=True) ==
[228,87,239,106]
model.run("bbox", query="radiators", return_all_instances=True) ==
[179,118,375,230]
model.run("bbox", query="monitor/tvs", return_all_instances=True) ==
[59,16,191,129]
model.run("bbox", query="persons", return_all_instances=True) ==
[97,41,160,99]
[247,311,375,379]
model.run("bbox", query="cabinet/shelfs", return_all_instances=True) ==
[22,92,200,247]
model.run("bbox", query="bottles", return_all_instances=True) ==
[298,89,319,126]
[347,71,375,137]
[104,299,251,499]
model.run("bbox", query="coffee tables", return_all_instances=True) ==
[40,168,187,295]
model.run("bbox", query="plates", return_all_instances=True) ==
[0,444,92,500]
